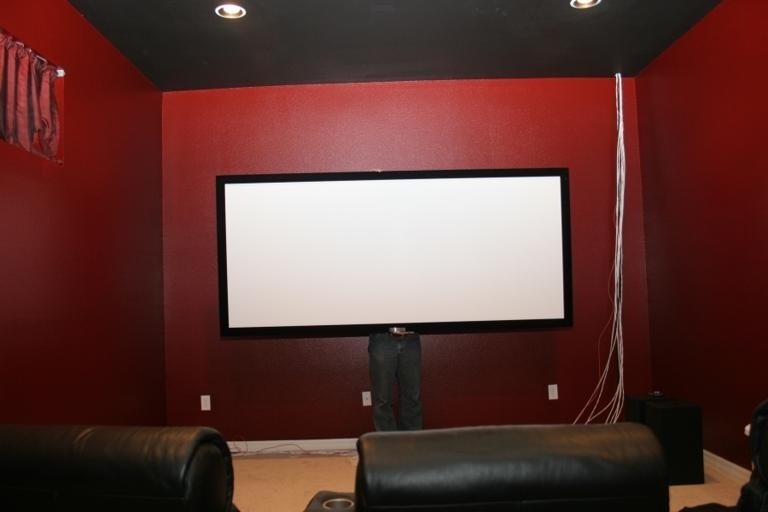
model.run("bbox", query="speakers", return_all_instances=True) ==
[623,391,665,422]
[642,398,705,487]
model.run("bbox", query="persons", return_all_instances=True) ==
[366,331,424,433]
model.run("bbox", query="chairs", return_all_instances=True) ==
[1,425,244,511]
[350,425,669,511]
